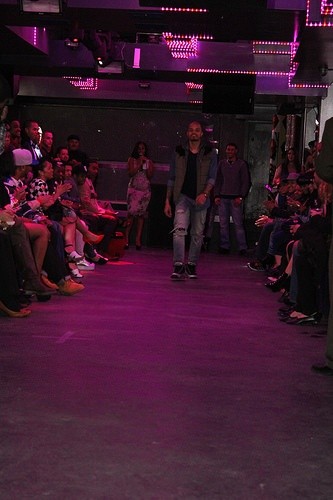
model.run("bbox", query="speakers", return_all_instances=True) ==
[201,72,256,115]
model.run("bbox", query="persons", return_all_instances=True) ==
[0,149,120,317]
[0,119,10,155]
[7,118,22,149]
[312,118,333,374]
[19,120,39,162]
[57,146,70,162]
[247,139,333,323]
[66,136,87,162]
[123,141,153,249]
[214,144,248,256]
[165,120,222,279]
[40,129,54,157]
[33,127,42,160]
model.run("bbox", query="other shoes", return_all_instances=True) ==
[75,257,95,271]
[124,245,129,249]
[89,234,105,244]
[204,240,211,252]
[185,264,197,277]
[16,275,58,295]
[104,252,119,261]
[57,275,85,295]
[217,247,224,255]
[171,265,185,278]
[311,365,333,376]
[136,245,141,250]
[241,249,320,326]
[67,251,82,261]
[89,250,109,264]
[71,269,83,278]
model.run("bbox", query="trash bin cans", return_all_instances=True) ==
[108,236,125,259]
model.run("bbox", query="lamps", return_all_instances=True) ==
[98,55,113,66]
[97,61,124,74]
[319,64,328,77]
[19,0,66,16]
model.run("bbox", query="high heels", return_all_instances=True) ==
[0,302,31,318]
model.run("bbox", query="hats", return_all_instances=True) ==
[13,149,33,165]
[286,173,300,180]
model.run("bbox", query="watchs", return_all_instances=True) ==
[238,197,243,201]
[202,192,208,198]
